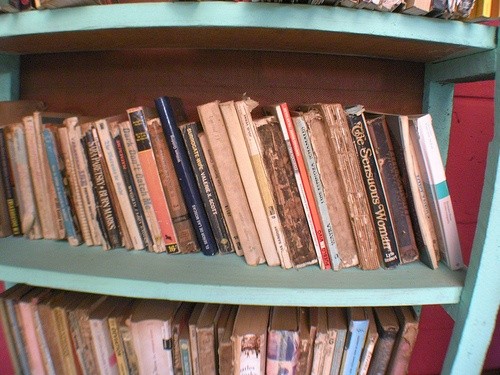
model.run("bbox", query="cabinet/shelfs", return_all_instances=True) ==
[1,1,500,375]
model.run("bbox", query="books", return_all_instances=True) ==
[0,282,423,375]
[0,91,467,275]
[0,0,499,22]
